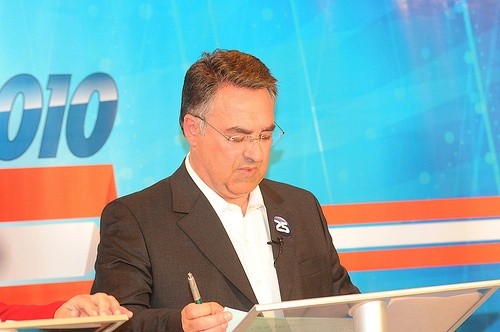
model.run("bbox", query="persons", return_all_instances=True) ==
[0,291,134,332]
[88,49,361,332]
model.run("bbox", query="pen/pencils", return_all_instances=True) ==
[187,271,203,305]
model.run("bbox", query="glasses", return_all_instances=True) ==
[194,115,285,144]
